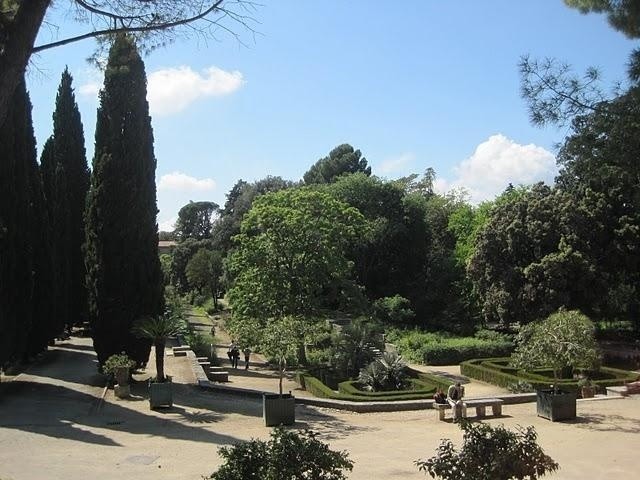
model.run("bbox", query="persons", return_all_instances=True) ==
[211,326,216,335]
[447,382,467,423]
[229,340,251,369]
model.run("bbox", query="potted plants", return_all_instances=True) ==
[231,312,350,427]
[509,308,604,421]
[102,354,135,398]
[128,315,190,409]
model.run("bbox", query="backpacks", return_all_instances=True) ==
[434,392,445,404]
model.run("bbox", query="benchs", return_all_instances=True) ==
[432,398,504,420]
